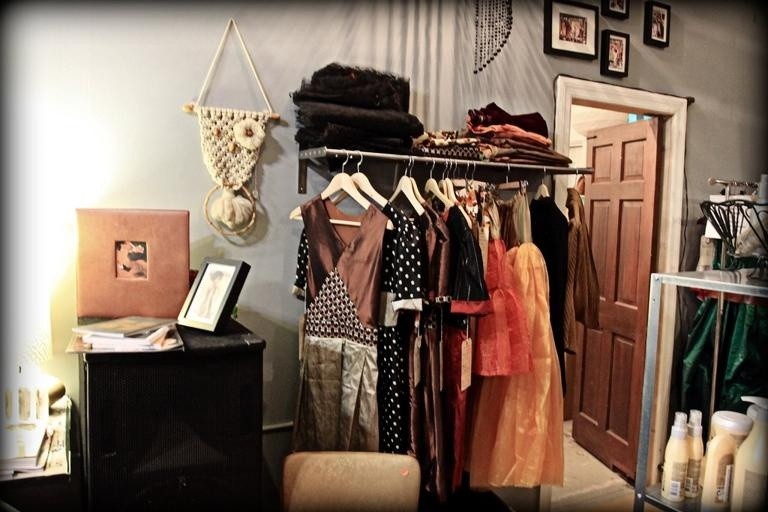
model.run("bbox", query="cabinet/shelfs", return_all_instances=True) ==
[632,268,768,512]
[79,316,266,512]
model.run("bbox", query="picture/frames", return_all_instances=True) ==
[600,30,629,78]
[174,257,251,336]
[544,0,598,60]
[642,0,671,48]
[602,0,630,20]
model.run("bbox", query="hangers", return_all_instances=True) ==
[730,181,758,195]
[289,149,580,231]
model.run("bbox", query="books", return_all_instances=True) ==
[0,419,54,479]
[72,315,177,350]
